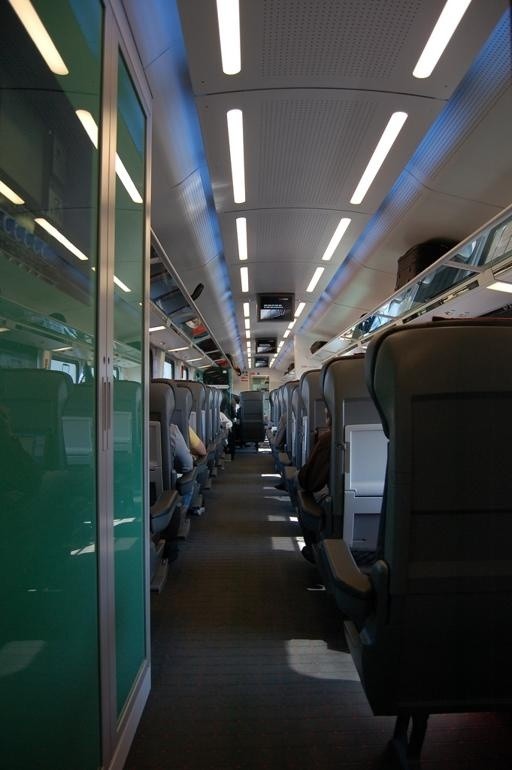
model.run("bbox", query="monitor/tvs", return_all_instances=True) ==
[254,336,279,354]
[257,292,296,323]
[254,356,269,367]
[203,366,230,386]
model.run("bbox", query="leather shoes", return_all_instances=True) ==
[275,485,286,490]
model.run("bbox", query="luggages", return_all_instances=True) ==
[394,244,465,303]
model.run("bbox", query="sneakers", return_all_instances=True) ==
[193,506,206,515]
[302,546,314,564]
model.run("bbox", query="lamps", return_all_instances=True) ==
[407,0,471,88]
[348,108,411,209]
[2,2,145,303]
[212,0,263,371]
[266,215,351,371]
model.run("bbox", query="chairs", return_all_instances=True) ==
[0,372,231,770]
[270,317,511,769]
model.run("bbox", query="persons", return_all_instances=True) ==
[219,412,234,431]
[168,422,197,517]
[232,395,241,425]
[273,412,290,492]
[188,426,207,517]
[294,408,333,565]
